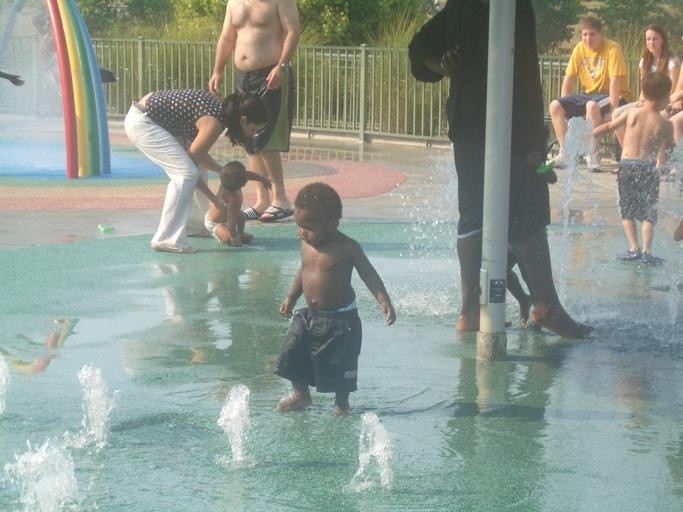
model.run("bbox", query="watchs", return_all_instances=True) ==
[281,61,289,71]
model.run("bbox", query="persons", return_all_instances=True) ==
[203,160,273,246]
[506,125,557,327]
[277,179,396,417]
[549,16,633,172]
[583,71,671,265]
[612,23,683,176]
[124,90,270,254]
[407,1,594,337]
[208,0,300,223]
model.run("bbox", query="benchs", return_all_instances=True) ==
[544,114,621,162]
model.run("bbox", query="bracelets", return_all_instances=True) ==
[536,164,548,172]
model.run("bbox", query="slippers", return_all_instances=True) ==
[242,207,261,220]
[262,205,293,221]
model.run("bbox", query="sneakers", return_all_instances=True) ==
[588,155,600,172]
[641,252,663,264]
[547,155,566,168]
[620,250,639,261]
